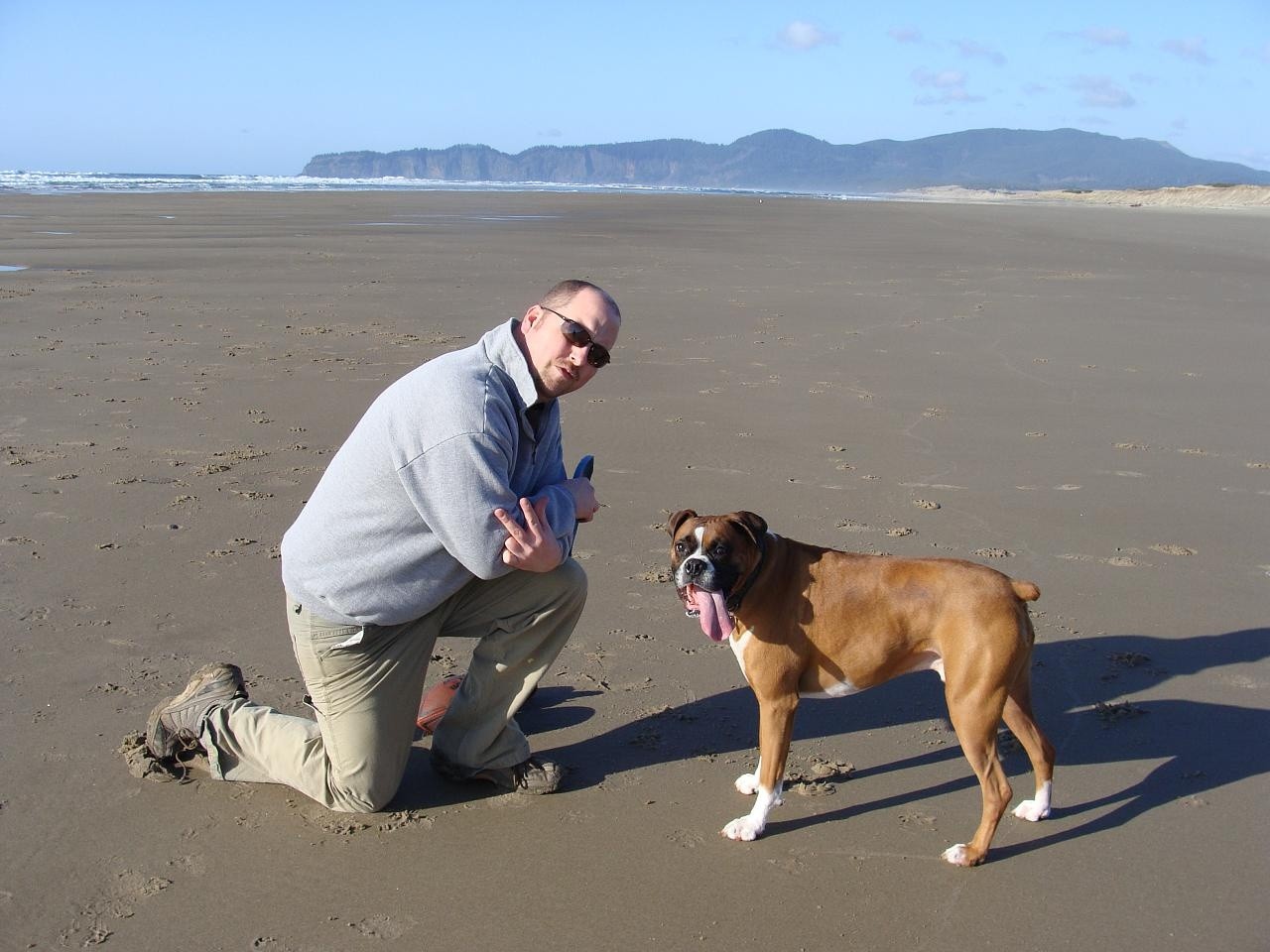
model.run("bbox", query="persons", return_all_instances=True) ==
[144,279,622,814]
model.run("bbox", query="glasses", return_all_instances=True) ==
[541,308,611,368]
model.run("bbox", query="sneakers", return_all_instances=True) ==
[145,663,248,784]
[430,742,569,795]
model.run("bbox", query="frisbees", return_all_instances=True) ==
[569,453,595,558]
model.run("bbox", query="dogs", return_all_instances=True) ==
[665,508,1056,867]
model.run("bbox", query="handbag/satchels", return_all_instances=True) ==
[413,675,539,742]
